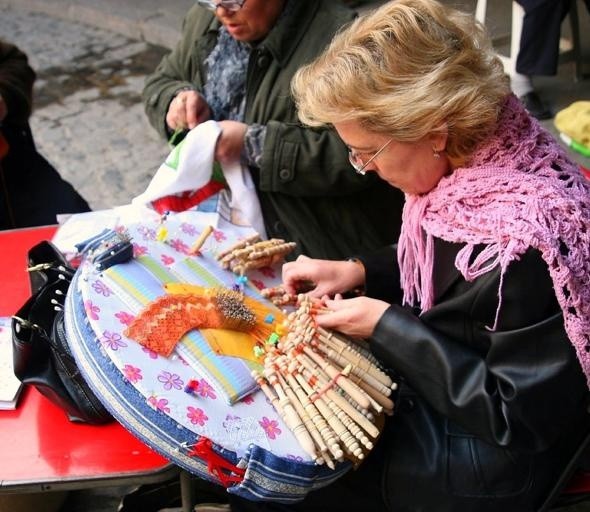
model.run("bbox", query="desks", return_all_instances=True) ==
[0,222,195,512]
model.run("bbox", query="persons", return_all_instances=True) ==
[135,0,395,261]
[508,1,574,121]
[0,42,94,231]
[227,1,590,511]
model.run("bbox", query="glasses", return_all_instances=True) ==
[198,0,244,12]
[348,138,394,177]
